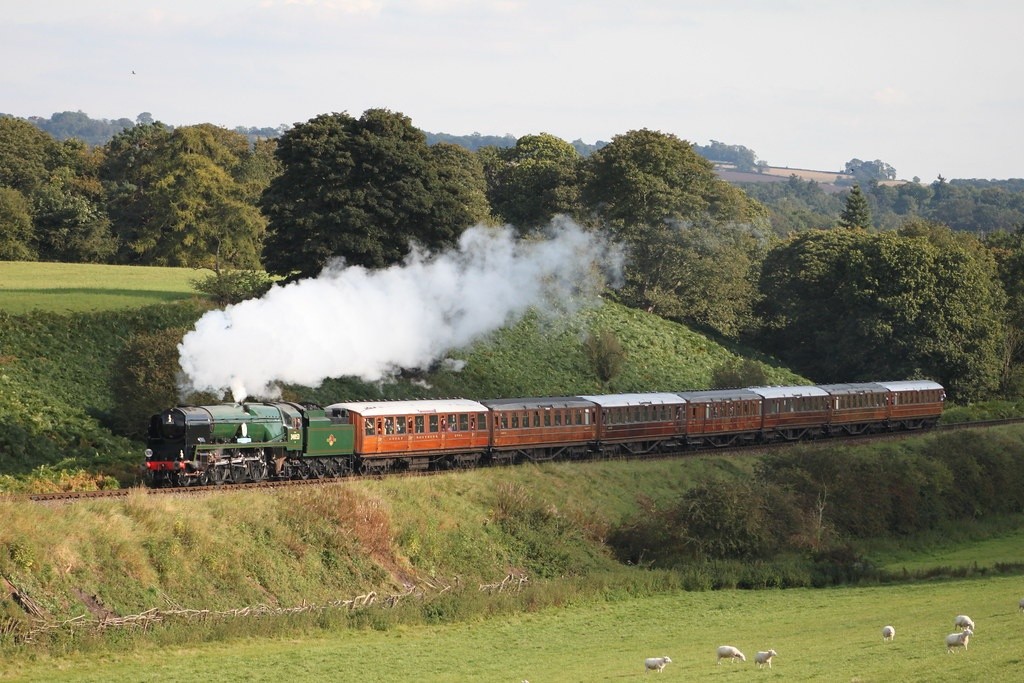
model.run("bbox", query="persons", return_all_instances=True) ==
[363,414,480,435]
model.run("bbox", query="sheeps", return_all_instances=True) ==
[954,615,974,630]
[946,628,973,653]
[717,646,746,664]
[753,649,777,669]
[883,626,895,641]
[1019,598,1024,611]
[645,656,671,674]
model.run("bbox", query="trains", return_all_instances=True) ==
[143,379,947,490]
